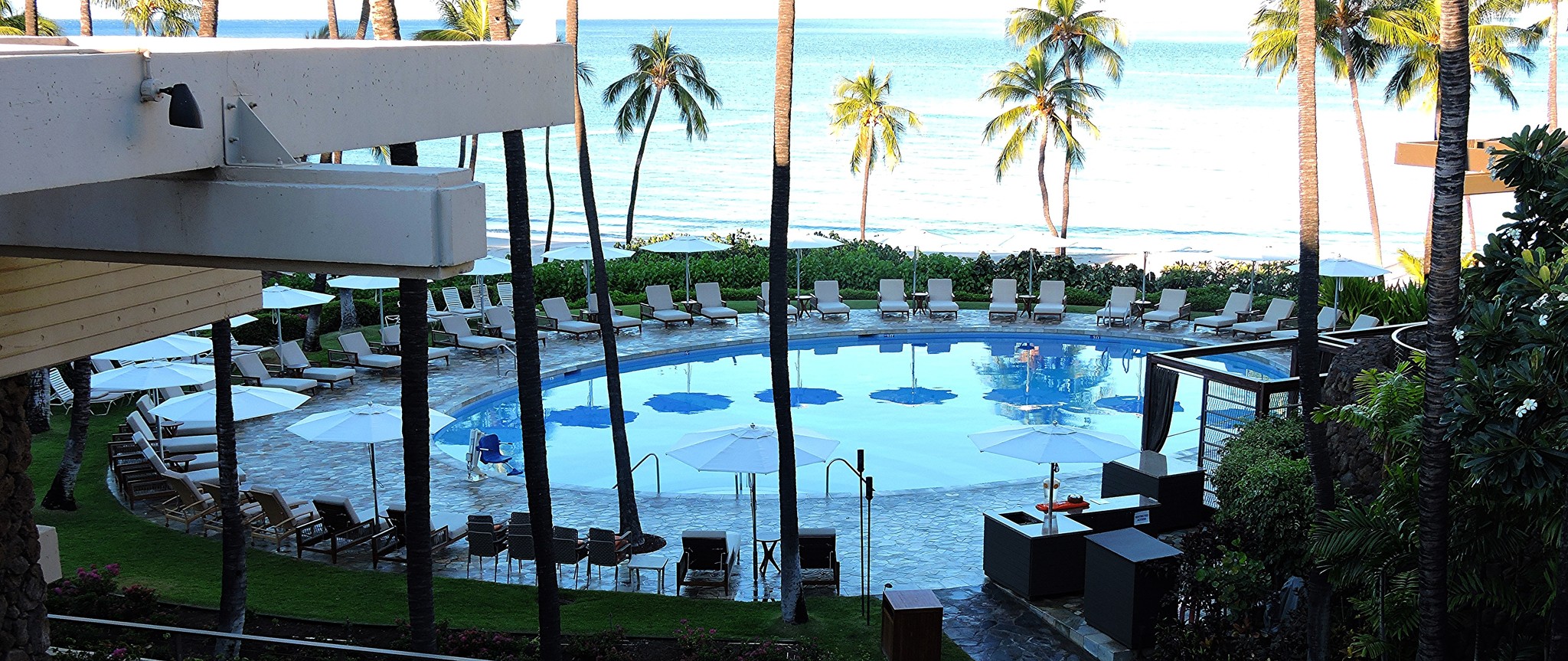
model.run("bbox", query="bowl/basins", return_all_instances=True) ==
[1067,495,1083,503]
[1067,510,1082,514]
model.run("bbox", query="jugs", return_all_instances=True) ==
[1042,515,1058,536]
[1044,478,1060,504]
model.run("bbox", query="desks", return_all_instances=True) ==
[1233,310,1254,336]
[751,532,782,577]
[911,292,929,316]
[1018,295,1036,319]
[794,295,813,319]
[1133,301,1151,324]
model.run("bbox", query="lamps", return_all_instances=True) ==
[138,76,204,129]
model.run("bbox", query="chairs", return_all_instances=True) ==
[1270,306,1344,349]
[988,278,1018,320]
[1343,314,1379,343]
[797,527,840,594]
[1096,285,1137,327]
[809,280,850,321]
[1232,298,1296,341]
[924,278,959,319]
[47,281,742,598]
[877,278,910,319]
[1033,280,1067,322]
[757,282,798,322]
[1142,289,1191,330]
[1193,292,1253,335]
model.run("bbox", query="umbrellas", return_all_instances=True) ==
[288,401,457,527]
[183,310,260,366]
[639,234,735,303]
[665,421,842,582]
[540,241,636,312]
[149,383,311,425]
[87,335,215,363]
[992,229,1079,295]
[967,419,1144,518]
[1214,243,1300,312]
[88,359,217,464]
[879,227,962,315]
[750,229,846,317]
[1285,253,1394,334]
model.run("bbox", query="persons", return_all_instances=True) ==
[455,255,512,327]
[251,282,336,371]
[325,273,435,345]
